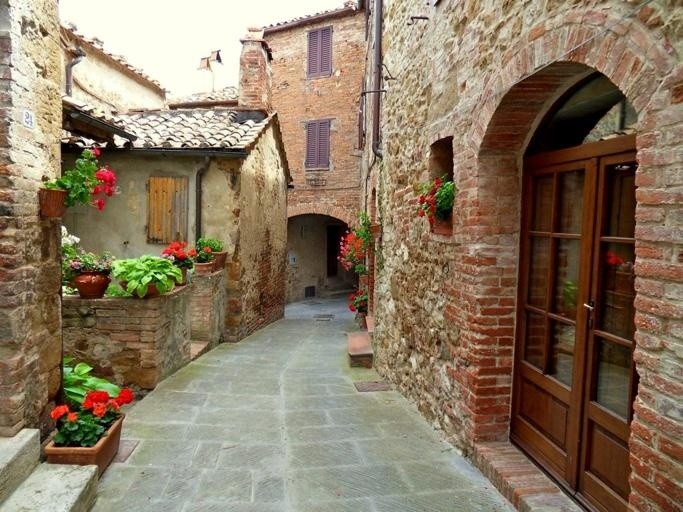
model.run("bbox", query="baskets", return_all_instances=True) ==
[370,223,383,238]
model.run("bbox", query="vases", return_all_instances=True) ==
[175,267,187,286]
[187,247,215,263]
[606,270,632,295]
[195,261,213,275]
[45,413,126,481]
[73,271,111,299]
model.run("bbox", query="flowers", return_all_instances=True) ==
[161,241,193,269]
[607,252,624,271]
[336,230,366,272]
[50,388,134,447]
[68,248,114,274]
[348,289,368,312]
[43,147,117,212]
[416,174,455,224]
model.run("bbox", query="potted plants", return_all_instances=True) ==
[196,238,228,271]
[111,254,183,299]
